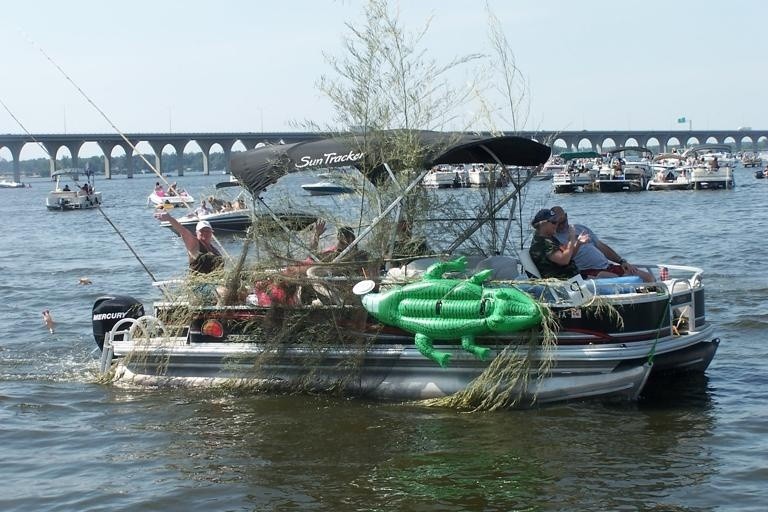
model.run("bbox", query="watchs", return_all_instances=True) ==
[619,258,625,263]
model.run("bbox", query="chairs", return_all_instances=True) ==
[520,249,543,282]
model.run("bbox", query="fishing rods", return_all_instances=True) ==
[39,49,237,270]
[0,102,172,304]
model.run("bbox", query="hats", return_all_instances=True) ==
[532,209,561,225]
[195,221,212,232]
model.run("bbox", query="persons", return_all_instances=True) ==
[431,147,768,184]
[152,208,225,300]
[59,180,247,217]
[550,206,653,284]
[284,218,357,275]
[528,208,591,279]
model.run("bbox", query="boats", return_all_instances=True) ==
[148,191,194,207]
[505,146,768,193]
[216,172,267,193]
[402,165,510,188]
[0,179,26,188]
[46,168,102,209]
[301,171,369,195]
[91,128,721,409]
[159,207,323,237]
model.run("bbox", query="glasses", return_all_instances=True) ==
[547,220,559,224]
